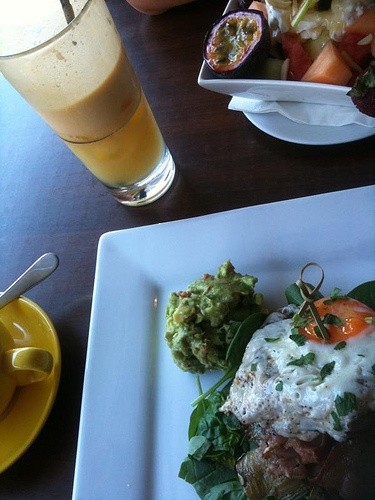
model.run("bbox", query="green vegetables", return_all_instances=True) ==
[177,284,359,500]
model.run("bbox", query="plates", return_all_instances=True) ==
[0,291,62,474]
[243,110,375,145]
[71,184,375,500]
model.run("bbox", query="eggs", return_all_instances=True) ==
[218,296,375,444]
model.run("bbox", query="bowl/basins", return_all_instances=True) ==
[198,0,359,107]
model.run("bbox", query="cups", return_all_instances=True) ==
[0,320,54,416]
[0,0,175,206]
